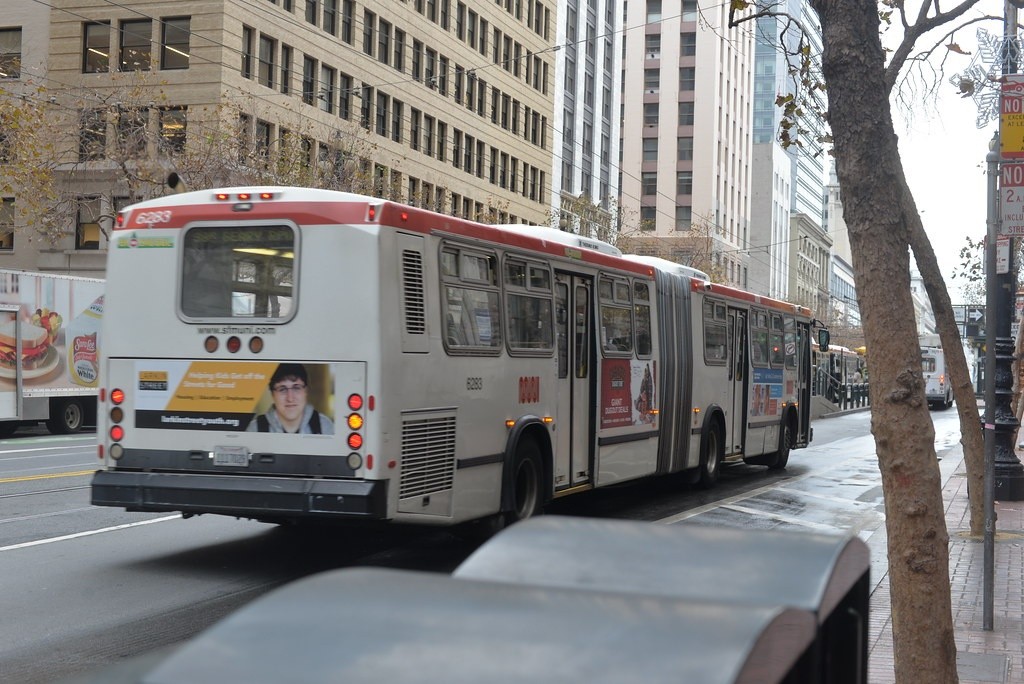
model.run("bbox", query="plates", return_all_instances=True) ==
[0,345,59,380]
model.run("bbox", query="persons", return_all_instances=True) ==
[850,367,863,409]
[762,385,772,415]
[245,363,334,435]
[751,385,764,416]
[634,363,654,424]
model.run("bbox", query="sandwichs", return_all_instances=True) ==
[0,320,51,369]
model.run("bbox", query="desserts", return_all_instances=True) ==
[32,308,62,344]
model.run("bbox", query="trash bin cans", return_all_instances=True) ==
[147,512,872,684]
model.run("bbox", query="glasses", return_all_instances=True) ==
[273,384,307,394]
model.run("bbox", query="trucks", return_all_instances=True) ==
[0,269,291,439]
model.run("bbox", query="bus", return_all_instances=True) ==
[919,347,978,411]
[88,186,830,550]
[811,343,858,402]
